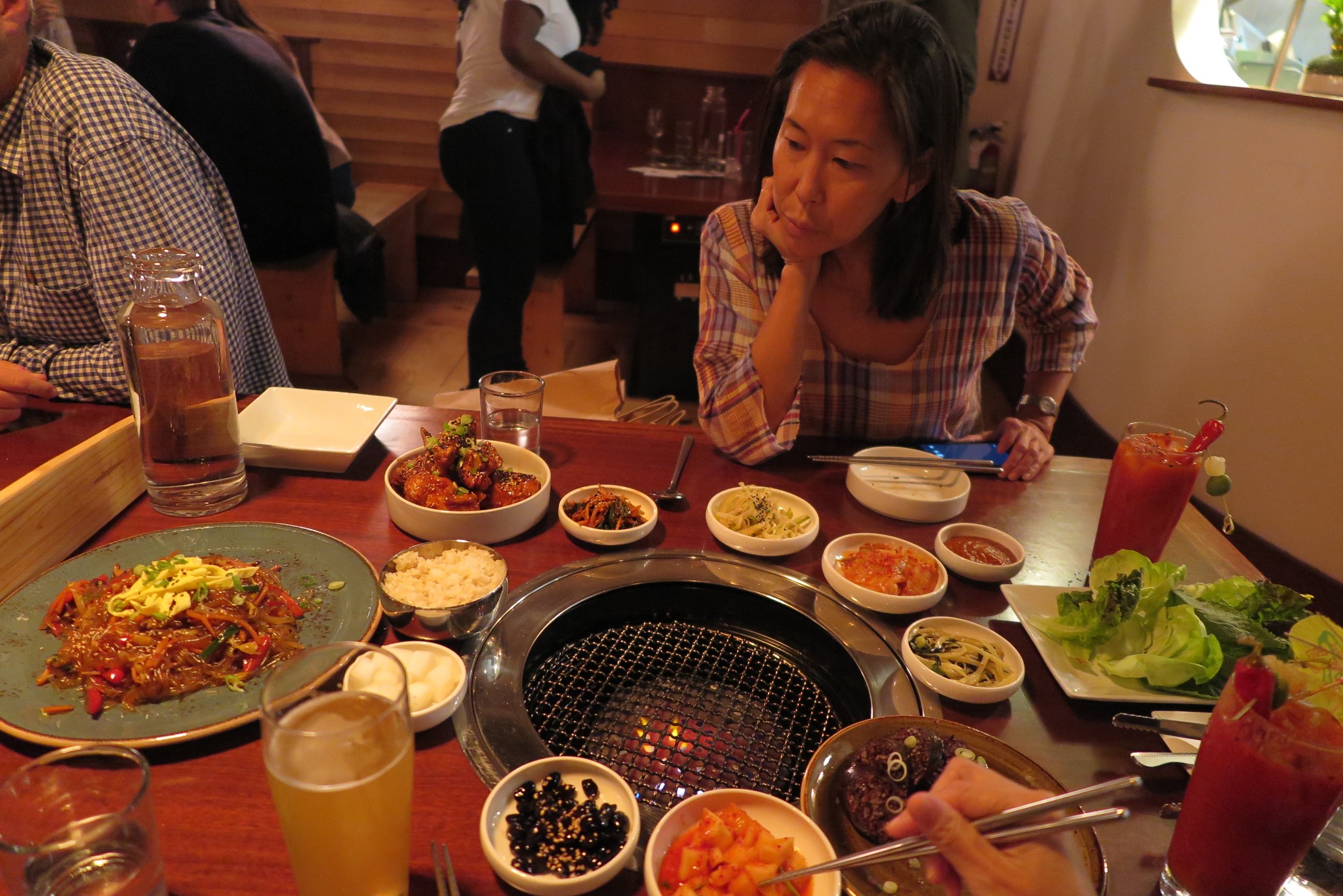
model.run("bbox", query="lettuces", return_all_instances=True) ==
[1029,545,1338,701]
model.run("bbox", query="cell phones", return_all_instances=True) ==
[913,438,1012,469]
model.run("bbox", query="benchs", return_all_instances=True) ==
[244,179,432,382]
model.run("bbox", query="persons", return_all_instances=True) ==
[0,0,303,405]
[212,1,361,215]
[696,1,1097,488]
[124,0,336,265]
[437,0,622,393]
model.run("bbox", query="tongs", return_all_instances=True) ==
[1112,714,1209,769]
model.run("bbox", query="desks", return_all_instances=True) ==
[551,140,786,316]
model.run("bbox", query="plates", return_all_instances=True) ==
[801,715,1108,896]
[846,446,970,523]
[558,484,657,545]
[822,532,948,614]
[1151,710,1214,775]
[480,756,640,896]
[342,640,469,736]
[999,583,1221,706]
[236,385,398,472]
[643,789,842,896]
[705,486,819,557]
[934,522,1025,582]
[901,616,1025,703]
[1,522,382,748]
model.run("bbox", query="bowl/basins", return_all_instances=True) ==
[385,438,551,544]
[377,539,508,644]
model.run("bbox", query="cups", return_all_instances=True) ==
[727,127,755,179]
[0,744,168,895]
[1082,421,1207,587]
[261,642,416,895]
[478,370,545,458]
[643,102,678,170]
[1159,672,1342,896]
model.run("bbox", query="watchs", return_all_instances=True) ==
[1020,391,1062,422]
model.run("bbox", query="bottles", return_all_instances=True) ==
[692,86,727,176]
[117,247,250,517]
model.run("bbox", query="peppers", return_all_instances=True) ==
[1233,634,1274,741]
[1176,398,1230,466]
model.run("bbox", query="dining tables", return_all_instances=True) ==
[2,391,1343,896]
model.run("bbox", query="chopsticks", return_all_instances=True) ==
[756,774,1144,887]
